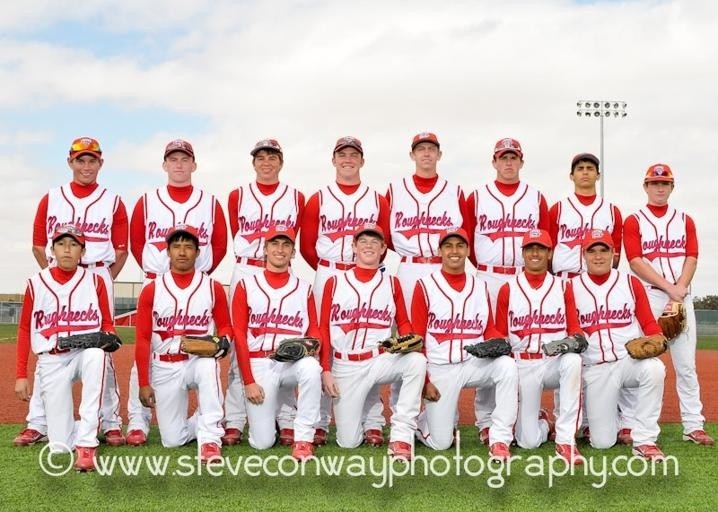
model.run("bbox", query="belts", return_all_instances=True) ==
[477,264,525,275]
[152,351,190,364]
[400,255,442,264]
[508,351,544,359]
[40,348,71,354]
[334,347,384,361]
[317,257,356,270]
[145,272,158,279]
[650,284,677,290]
[248,350,275,359]
[235,255,292,268]
[556,272,581,279]
[48,257,105,269]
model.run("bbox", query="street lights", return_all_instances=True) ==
[575,98,628,197]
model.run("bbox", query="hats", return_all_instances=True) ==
[164,138,194,160]
[52,225,85,246]
[571,152,599,166]
[334,136,362,155]
[166,223,199,243]
[495,137,523,159]
[266,224,296,244]
[643,163,674,183]
[438,228,469,247]
[521,228,553,249]
[582,228,615,253]
[354,223,384,243]
[69,137,102,162]
[412,132,438,150]
[250,139,282,157]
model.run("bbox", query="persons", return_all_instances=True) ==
[222,140,305,446]
[126,140,227,447]
[549,154,623,442]
[300,138,390,444]
[466,138,549,444]
[231,224,322,462]
[14,224,116,472]
[14,138,126,447]
[618,164,714,446]
[495,229,587,464]
[411,227,518,463]
[321,222,427,460]
[570,229,666,463]
[135,224,234,465]
[384,133,466,437]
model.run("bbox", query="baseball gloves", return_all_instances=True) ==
[269,337,319,362]
[463,338,511,358]
[180,336,230,359]
[56,332,121,352]
[541,334,587,356]
[624,335,667,359]
[382,334,423,353]
[657,303,685,341]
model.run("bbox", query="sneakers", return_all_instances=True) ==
[488,442,511,465]
[630,444,665,463]
[292,440,313,463]
[74,447,98,472]
[12,428,48,446]
[554,442,586,465]
[479,410,635,446]
[128,430,146,446]
[105,429,126,446]
[364,428,383,446]
[682,429,713,446]
[200,441,222,463]
[312,426,327,446]
[386,440,413,462]
[280,425,294,446]
[222,428,243,445]
